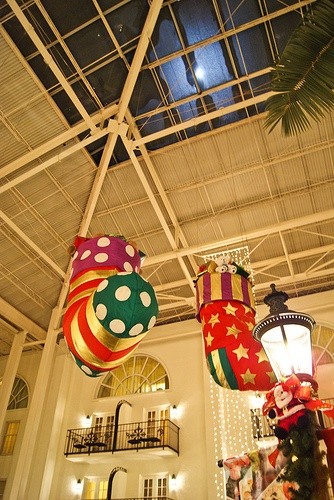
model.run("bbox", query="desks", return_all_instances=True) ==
[84,443,96,451]
[138,439,151,447]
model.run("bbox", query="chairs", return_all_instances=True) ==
[128,433,141,448]
[149,429,162,447]
[95,436,108,451]
[73,437,86,452]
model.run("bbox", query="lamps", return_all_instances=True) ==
[247,283,317,393]
